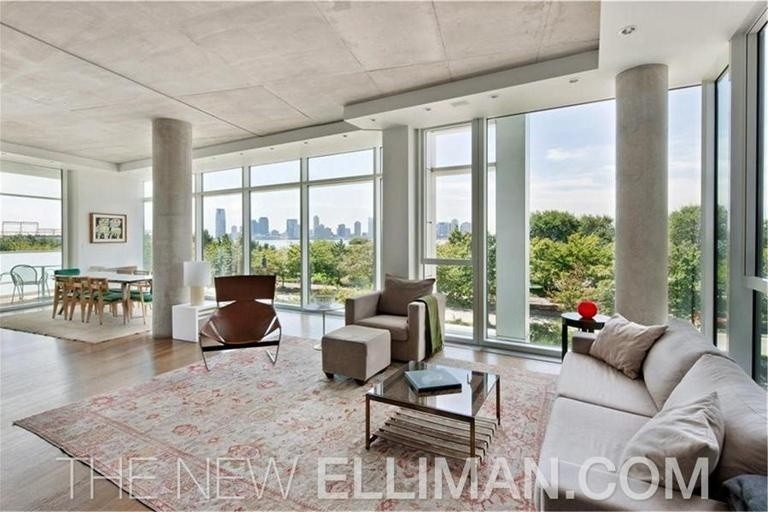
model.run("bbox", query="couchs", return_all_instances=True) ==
[537,317,768,512]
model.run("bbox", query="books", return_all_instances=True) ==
[405,367,463,394]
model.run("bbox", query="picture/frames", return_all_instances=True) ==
[89,213,127,243]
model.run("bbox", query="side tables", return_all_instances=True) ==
[303,303,345,351]
[561,312,612,363]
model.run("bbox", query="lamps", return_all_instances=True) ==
[184,261,210,306]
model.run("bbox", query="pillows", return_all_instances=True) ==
[588,313,669,380]
[616,392,725,492]
[723,473,768,512]
[641,318,738,413]
[659,353,768,497]
[378,273,436,317]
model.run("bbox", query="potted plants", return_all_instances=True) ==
[312,287,334,307]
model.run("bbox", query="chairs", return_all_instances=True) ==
[198,275,282,372]
[345,290,446,362]
[0,264,153,324]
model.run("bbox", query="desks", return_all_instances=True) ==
[172,300,230,344]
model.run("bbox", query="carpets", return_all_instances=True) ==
[12,333,561,512]
[0,304,152,344]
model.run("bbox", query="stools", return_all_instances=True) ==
[322,325,391,386]
